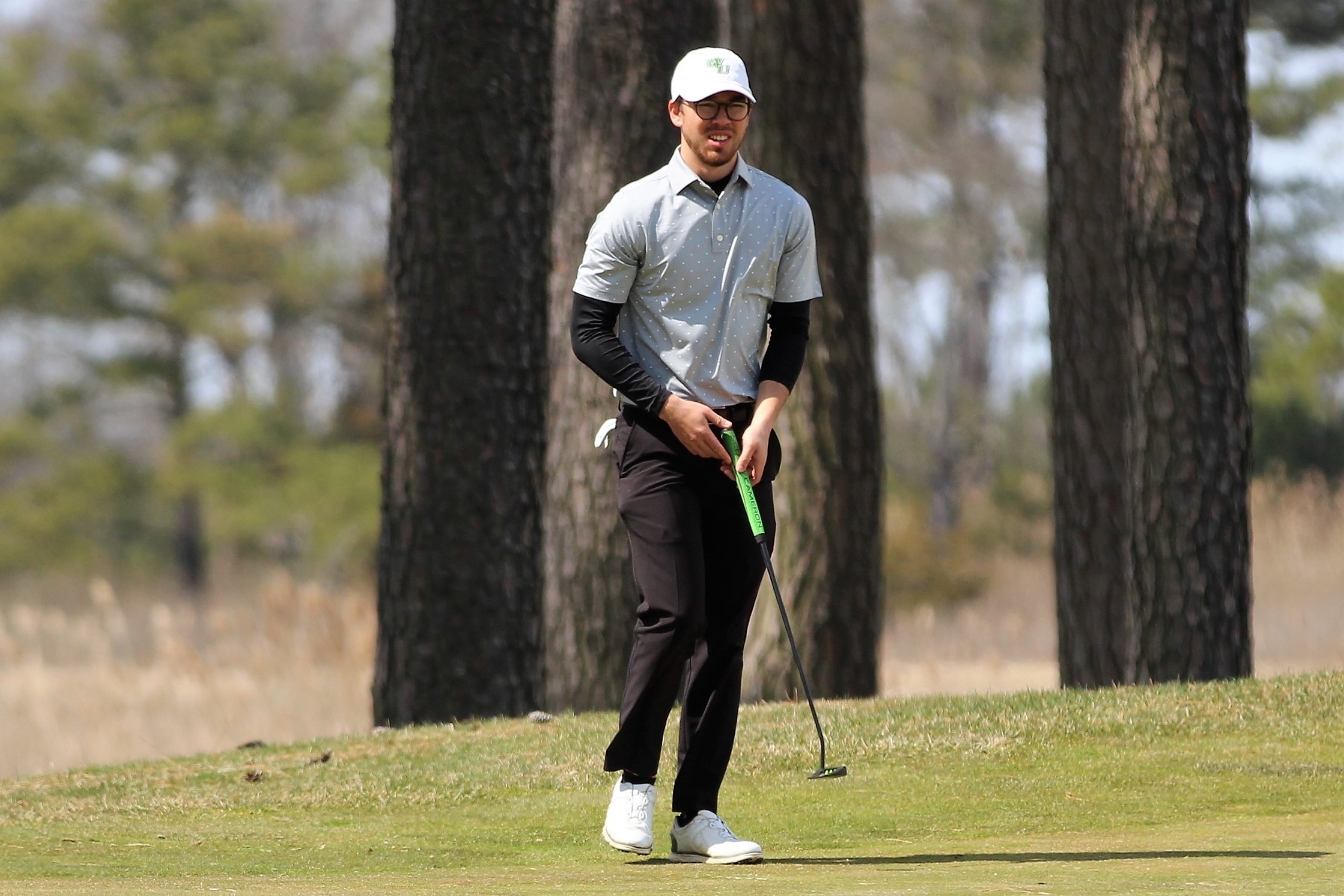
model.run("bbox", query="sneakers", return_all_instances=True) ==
[668,809,763,864]
[601,777,657,855]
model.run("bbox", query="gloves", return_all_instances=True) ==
[593,418,616,448]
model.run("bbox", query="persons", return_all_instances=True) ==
[572,47,825,865]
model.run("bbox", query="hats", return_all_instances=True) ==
[670,47,756,103]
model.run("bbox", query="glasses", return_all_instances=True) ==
[684,100,750,121]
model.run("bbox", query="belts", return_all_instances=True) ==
[706,407,750,425]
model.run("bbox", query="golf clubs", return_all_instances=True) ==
[718,425,847,779]
